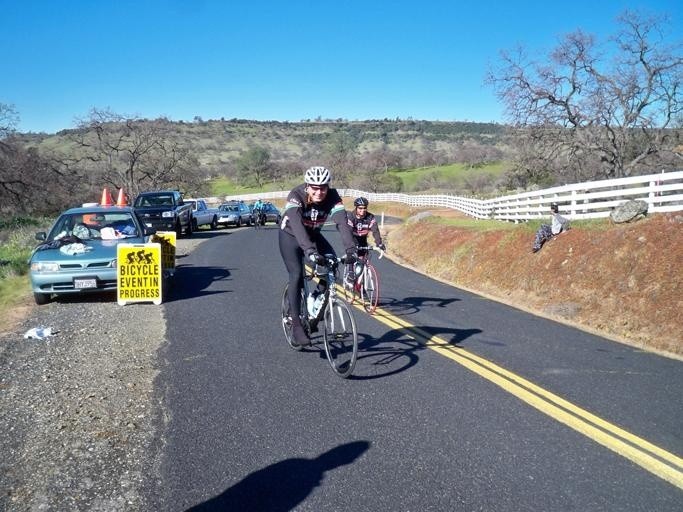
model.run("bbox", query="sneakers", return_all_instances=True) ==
[291,317,311,345]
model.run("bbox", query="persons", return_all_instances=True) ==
[253,199,265,223]
[532,202,567,252]
[277,166,357,347]
[344,196,386,286]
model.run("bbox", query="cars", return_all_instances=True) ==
[30,207,149,303]
[217,200,253,227]
[248,201,282,225]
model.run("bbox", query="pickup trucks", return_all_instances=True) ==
[134,190,193,239]
[183,199,220,231]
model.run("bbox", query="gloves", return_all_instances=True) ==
[375,238,386,251]
[304,242,325,266]
[345,247,355,254]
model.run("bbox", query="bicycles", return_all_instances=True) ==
[343,245,385,314]
[280,254,359,380]
[253,208,266,230]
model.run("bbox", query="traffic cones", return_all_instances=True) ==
[116,189,128,206]
[99,189,113,208]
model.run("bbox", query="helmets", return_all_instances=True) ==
[304,166,332,186]
[354,197,369,206]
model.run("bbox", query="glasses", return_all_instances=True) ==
[357,206,367,209]
[309,185,327,191]
